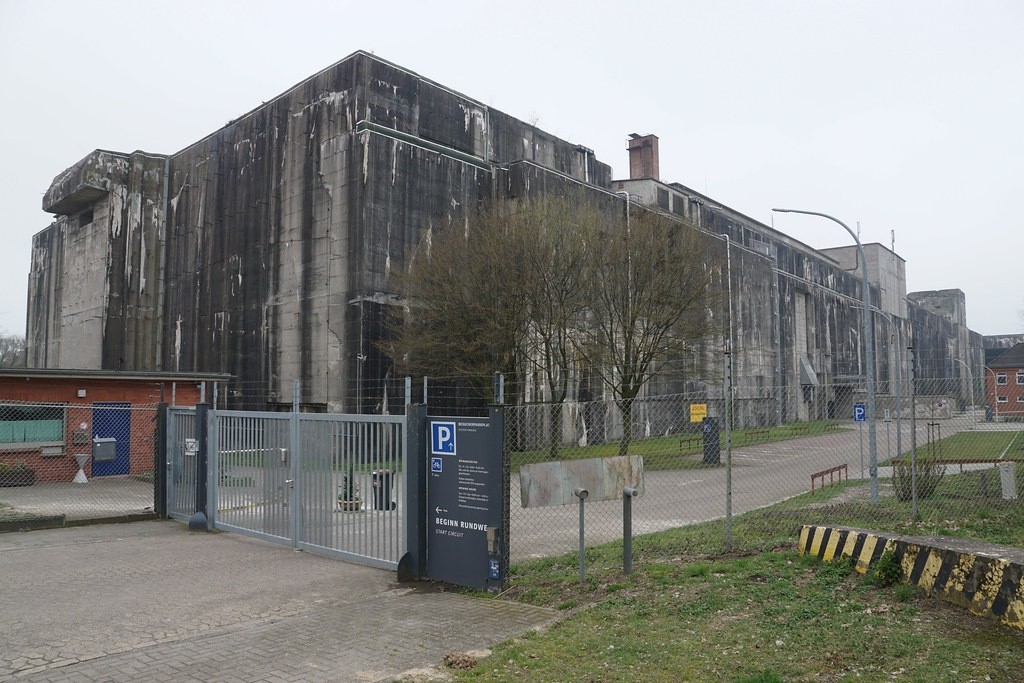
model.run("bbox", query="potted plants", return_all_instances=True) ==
[338,463,363,511]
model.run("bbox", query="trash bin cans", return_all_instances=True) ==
[702,417,723,463]
[985,405,992,420]
[369,469,396,509]
